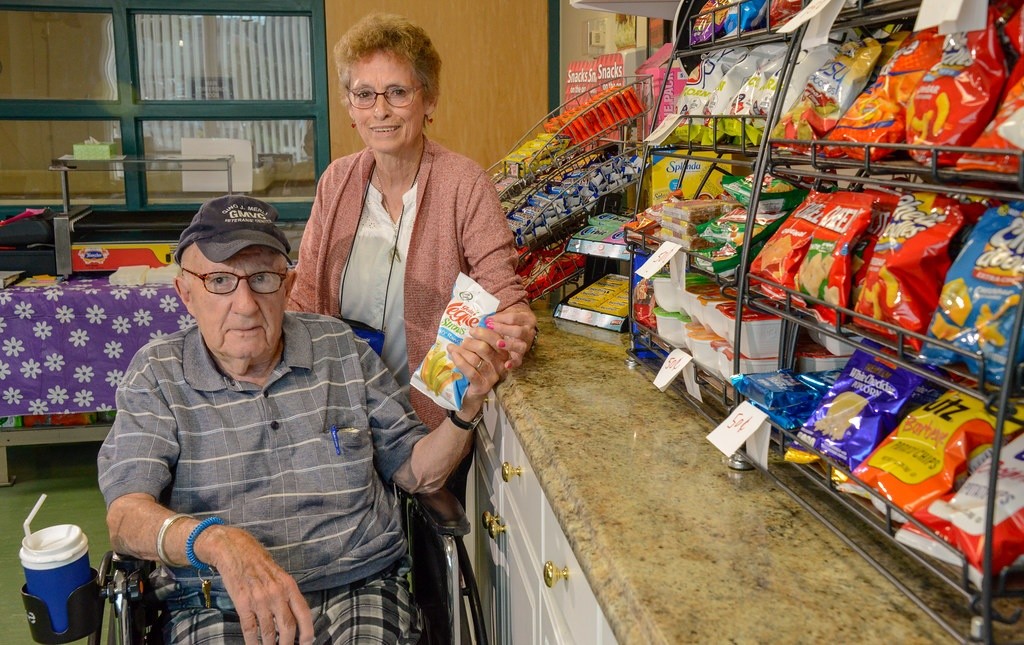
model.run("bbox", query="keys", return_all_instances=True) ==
[197,565,215,610]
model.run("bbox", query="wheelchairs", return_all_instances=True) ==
[21,480,495,645]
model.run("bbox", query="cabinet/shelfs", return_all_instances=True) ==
[51,151,236,279]
[473,0,1024,645]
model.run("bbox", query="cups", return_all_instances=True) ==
[20,521,91,631]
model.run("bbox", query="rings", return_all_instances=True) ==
[473,360,486,371]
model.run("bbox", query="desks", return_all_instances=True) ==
[0,275,197,487]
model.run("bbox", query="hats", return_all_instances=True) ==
[176,195,295,266]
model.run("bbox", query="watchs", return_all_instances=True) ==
[446,405,485,433]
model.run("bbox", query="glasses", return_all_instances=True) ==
[344,86,424,110]
[181,268,286,295]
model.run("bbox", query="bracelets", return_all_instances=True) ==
[528,326,543,360]
[153,509,197,572]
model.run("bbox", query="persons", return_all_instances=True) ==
[284,10,526,638]
[85,189,512,645]
